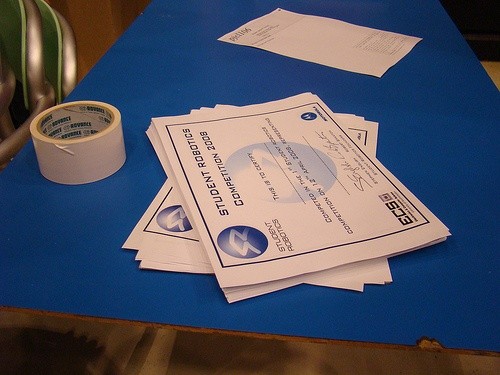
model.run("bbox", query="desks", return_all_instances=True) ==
[1,0,500,374]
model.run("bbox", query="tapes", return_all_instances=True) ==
[29,100,126,187]
